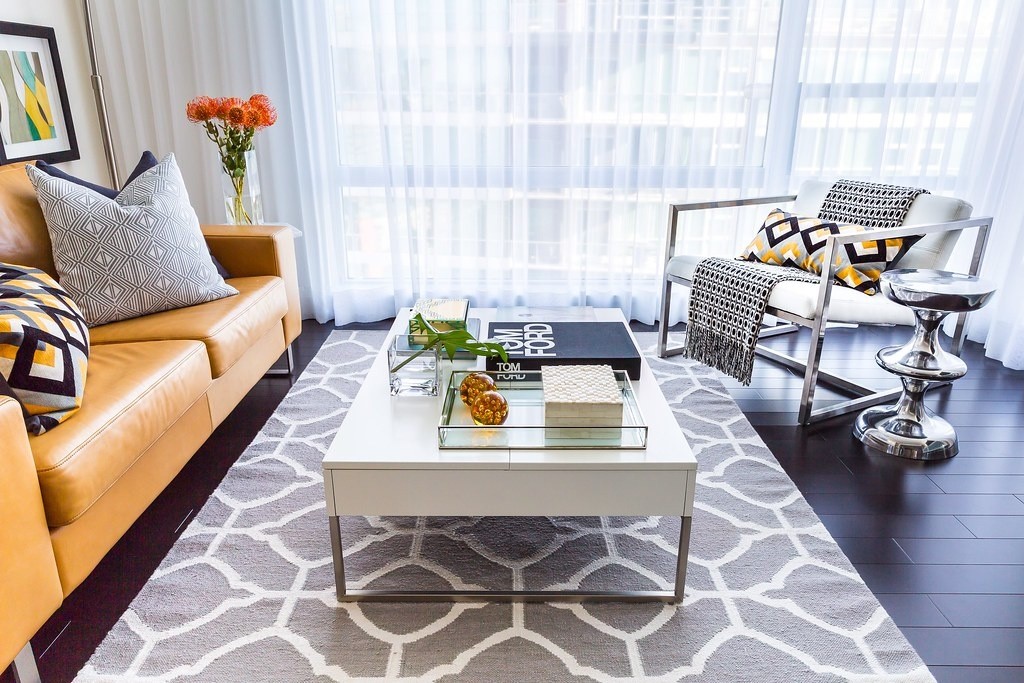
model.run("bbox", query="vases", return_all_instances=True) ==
[217,150,265,229]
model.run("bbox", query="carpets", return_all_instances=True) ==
[68,330,936,683]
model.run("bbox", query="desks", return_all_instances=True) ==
[323,306,698,604]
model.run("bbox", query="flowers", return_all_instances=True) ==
[186,93,278,225]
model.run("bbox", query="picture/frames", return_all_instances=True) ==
[0,20,81,163]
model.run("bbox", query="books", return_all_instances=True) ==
[388,317,480,361]
[486,322,642,382]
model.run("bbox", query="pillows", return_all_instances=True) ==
[0,262,91,436]
[35,150,229,280]
[24,153,239,330]
[734,207,904,296]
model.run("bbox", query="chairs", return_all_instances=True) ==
[656,179,994,427]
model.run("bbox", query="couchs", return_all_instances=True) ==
[0,159,301,683]
